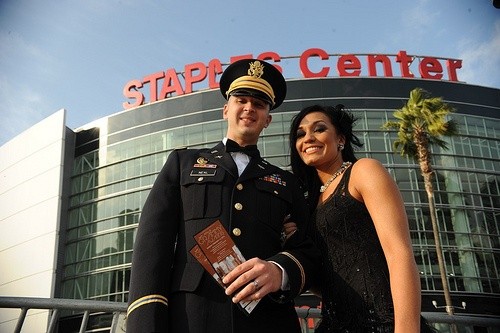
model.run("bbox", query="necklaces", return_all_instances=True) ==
[320,161,352,192]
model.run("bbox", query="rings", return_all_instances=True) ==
[280,232,286,239]
[252,281,259,290]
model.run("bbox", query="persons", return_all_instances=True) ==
[125,59,324,333]
[281,104,443,333]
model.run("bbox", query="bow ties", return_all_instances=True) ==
[225,137,257,158]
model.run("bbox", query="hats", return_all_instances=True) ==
[219,57,287,110]
